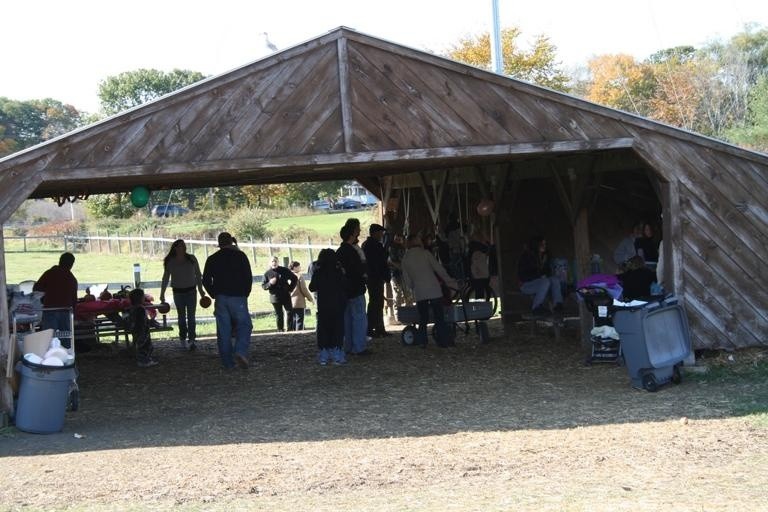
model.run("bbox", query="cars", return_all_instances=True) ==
[311,200,330,211]
[333,197,361,210]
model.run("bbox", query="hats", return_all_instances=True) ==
[368,223,389,233]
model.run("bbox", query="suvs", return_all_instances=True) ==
[150,204,190,218]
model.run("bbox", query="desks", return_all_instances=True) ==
[74,299,167,313]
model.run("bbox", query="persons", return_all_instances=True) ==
[261,256,312,331]
[336,218,395,353]
[309,249,351,367]
[33,253,78,349]
[162,240,204,348]
[517,236,565,317]
[389,225,495,348]
[203,232,253,369]
[129,289,158,367]
[614,219,661,299]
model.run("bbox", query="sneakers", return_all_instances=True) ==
[332,348,349,365]
[233,349,251,371]
[318,347,331,366]
[137,359,160,370]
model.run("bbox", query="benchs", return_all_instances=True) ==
[499,289,579,340]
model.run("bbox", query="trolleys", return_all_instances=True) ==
[397,283,496,348]
[10,305,80,412]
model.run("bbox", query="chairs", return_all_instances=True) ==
[90,317,174,348]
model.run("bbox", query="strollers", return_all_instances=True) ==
[579,288,626,369]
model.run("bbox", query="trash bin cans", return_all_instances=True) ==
[607,290,690,392]
[14,355,77,434]
